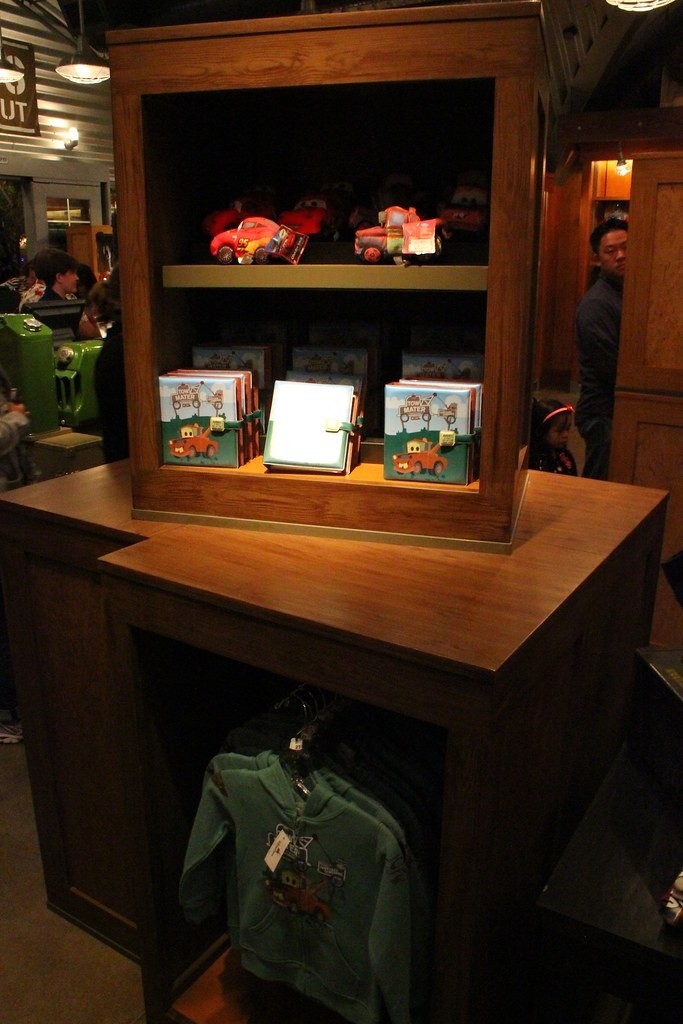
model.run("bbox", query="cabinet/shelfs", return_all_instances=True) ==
[106,5,560,555]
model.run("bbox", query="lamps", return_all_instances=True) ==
[54,1,111,86]
[1,48,26,84]
[614,150,632,179]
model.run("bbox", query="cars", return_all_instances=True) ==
[280,196,340,240]
[355,205,442,266]
[442,185,489,233]
[393,440,448,476]
[207,201,275,233]
[272,871,332,925]
[307,354,331,371]
[210,217,280,264]
[168,425,218,460]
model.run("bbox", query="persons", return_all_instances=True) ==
[0,396,41,743]
[574,218,629,477]
[529,400,577,476]
[0,247,101,339]
[93,263,130,463]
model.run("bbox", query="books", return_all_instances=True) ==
[157,346,271,467]
[382,352,483,486]
[263,348,370,476]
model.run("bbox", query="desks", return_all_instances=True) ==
[0,453,671,1023]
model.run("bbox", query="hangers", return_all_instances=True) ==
[271,678,343,771]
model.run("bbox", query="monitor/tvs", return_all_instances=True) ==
[20,298,89,351]
